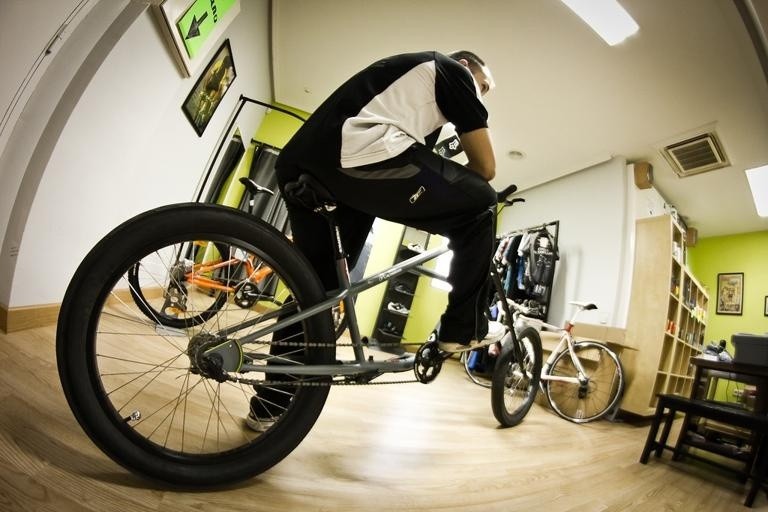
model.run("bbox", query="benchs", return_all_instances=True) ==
[639,392,768,508]
[525,331,639,421]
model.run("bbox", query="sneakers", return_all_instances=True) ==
[437,320,508,353]
[407,242,427,254]
[378,321,402,338]
[245,410,284,432]
[394,280,415,296]
[388,301,410,315]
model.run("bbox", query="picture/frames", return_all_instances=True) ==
[764,296,768,317]
[716,272,744,315]
[179,38,237,136]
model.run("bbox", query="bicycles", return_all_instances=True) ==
[128,178,350,340]
[55,174,542,481]
[464,297,625,423]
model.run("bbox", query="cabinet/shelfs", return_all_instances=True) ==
[370,225,431,353]
[603,214,710,423]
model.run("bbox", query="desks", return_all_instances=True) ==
[671,353,767,496]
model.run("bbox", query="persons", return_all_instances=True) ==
[241,48,499,438]
